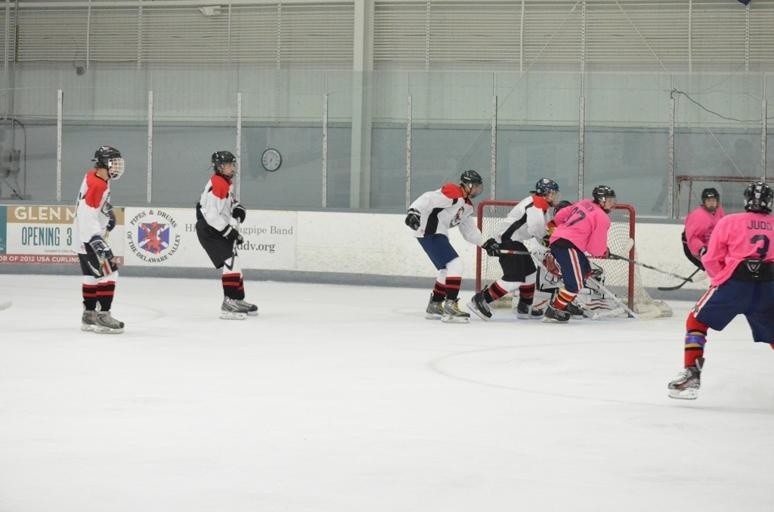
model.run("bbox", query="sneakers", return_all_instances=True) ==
[544,305,570,321]
[82,310,98,324]
[565,301,583,315]
[94,311,124,329]
[236,299,257,312]
[221,296,246,312]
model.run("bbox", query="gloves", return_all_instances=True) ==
[481,238,501,256]
[220,224,243,244]
[106,210,116,231]
[232,204,246,222]
[405,208,421,230]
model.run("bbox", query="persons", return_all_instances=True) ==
[194,151,258,313]
[69,145,126,330]
[667,181,773,391]
[535,198,604,312]
[467,178,558,320]
[681,188,726,272]
[403,170,497,318]
[545,184,616,321]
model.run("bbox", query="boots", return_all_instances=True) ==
[443,297,470,318]
[518,294,543,315]
[471,285,492,318]
[668,356,704,391]
[426,292,444,316]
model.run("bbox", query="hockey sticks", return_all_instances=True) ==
[85,223,112,277]
[657,269,700,290]
[220,217,241,270]
[574,296,624,318]
[499,238,635,260]
[587,276,658,319]
[610,254,694,284]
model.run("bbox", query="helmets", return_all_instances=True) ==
[593,185,616,209]
[460,170,483,198]
[702,188,719,211]
[743,180,774,214]
[536,177,560,202]
[95,145,124,181]
[212,150,239,179]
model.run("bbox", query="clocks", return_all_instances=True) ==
[260,147,281,172]
